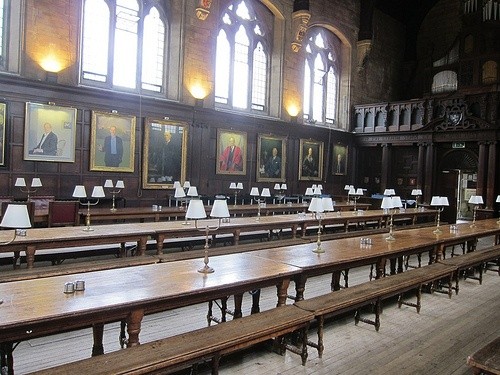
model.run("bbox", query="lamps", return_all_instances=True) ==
[291,116,298,123]
[46,71,58,84]
[196,99,203,108]
[0,177,500,274]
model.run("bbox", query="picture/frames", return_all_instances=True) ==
[0,101,348,190]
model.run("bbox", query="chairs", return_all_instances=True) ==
[47,201,80,228]
[2,202,35,226]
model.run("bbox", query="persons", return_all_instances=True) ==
[357,177,372,210]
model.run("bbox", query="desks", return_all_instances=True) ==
[365,207,439,229]
[184,204,268,220]
[274,210,382,237]
[132,214,303,256]
[332,202,371,212]
[267,203,310,215]
[80,205,185,225]
[0,224,156,270]
[467,335,500,375]
[249,232,440,314]
[0,253,303,375]
[387,224,500,273]
[466,217,500,253]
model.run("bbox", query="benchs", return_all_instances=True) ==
[0,220,500,375]
